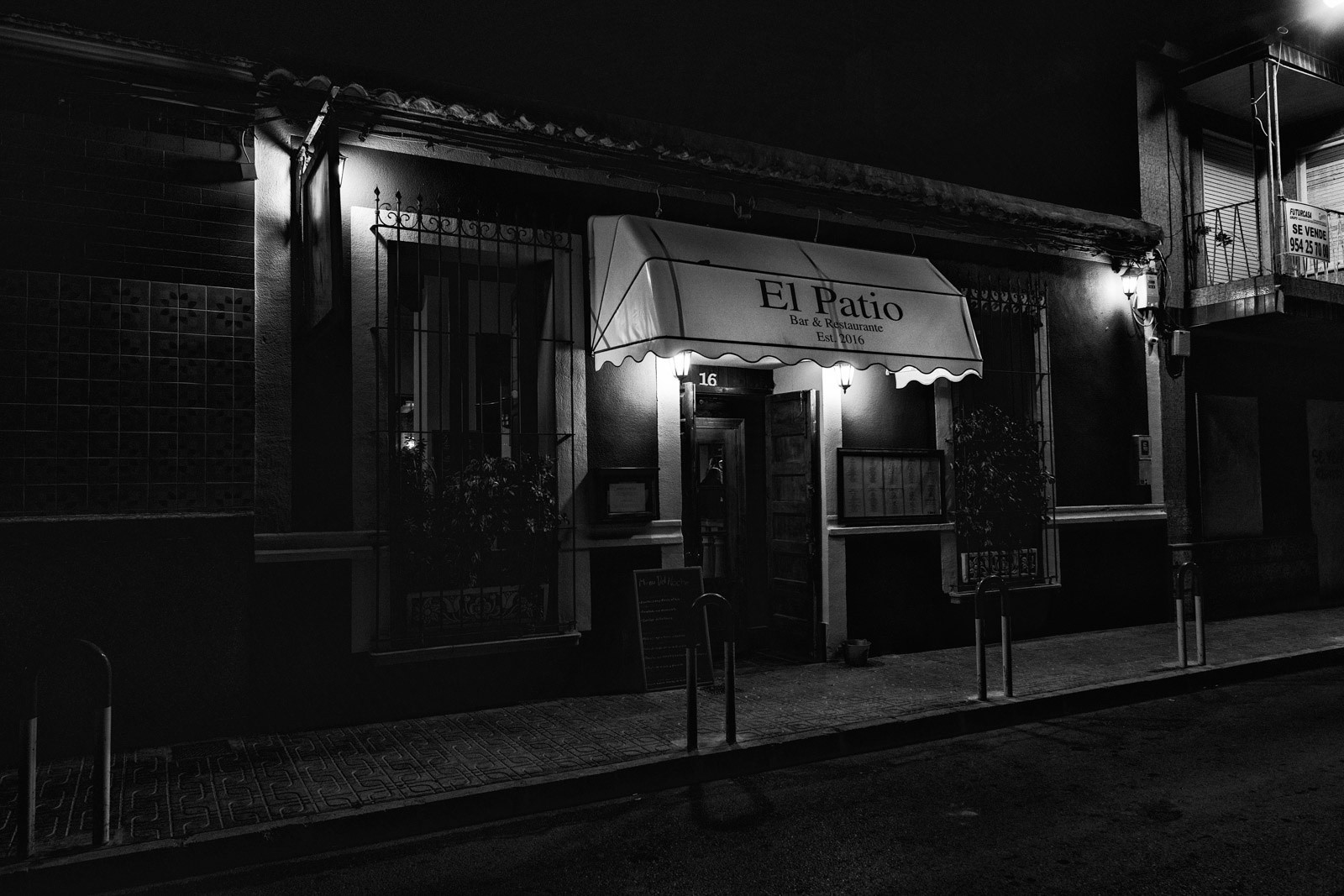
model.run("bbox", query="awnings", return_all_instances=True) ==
[587,214,983,390]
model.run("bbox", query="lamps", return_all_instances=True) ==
[674,352,691,385]
[1120,265,1139,300]
[833,363,855,394]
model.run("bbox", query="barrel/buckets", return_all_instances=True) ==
[840,638,871,668]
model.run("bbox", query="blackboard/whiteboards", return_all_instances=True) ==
[635,566,714,690]
